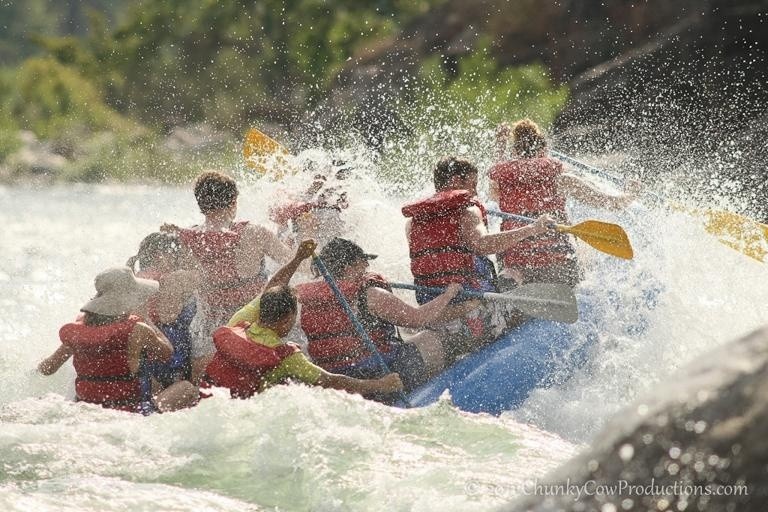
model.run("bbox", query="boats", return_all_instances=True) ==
[384,198,665,418]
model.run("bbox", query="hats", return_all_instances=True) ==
[79,266,160,319]
[311,237,378,265]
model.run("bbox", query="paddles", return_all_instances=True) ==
[550,150,768,265]
[486,209,633,259]
[389,283,578,324]
[244,129,293,174]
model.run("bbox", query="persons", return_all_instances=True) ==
[37,117,645,416]
